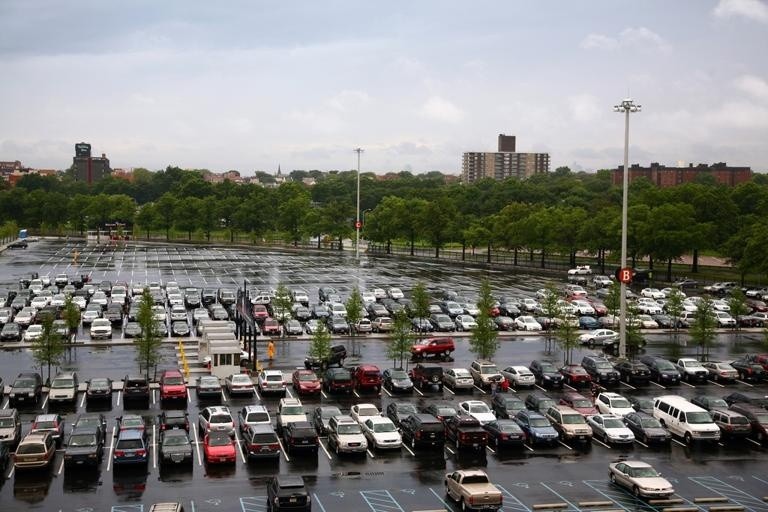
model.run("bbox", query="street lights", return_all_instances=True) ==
[353,144,365,259]
[611,98,643,362]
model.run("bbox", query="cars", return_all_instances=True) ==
[567,265,593,276]
[21,236,41,243]
[607,460,676,499]
[7,242,28,249]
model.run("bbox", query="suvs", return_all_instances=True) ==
[265,472,312,512]
[147,502,185,512]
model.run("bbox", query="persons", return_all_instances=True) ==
[268,341,274,365]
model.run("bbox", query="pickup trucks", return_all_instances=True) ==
[445,468,505,512]
